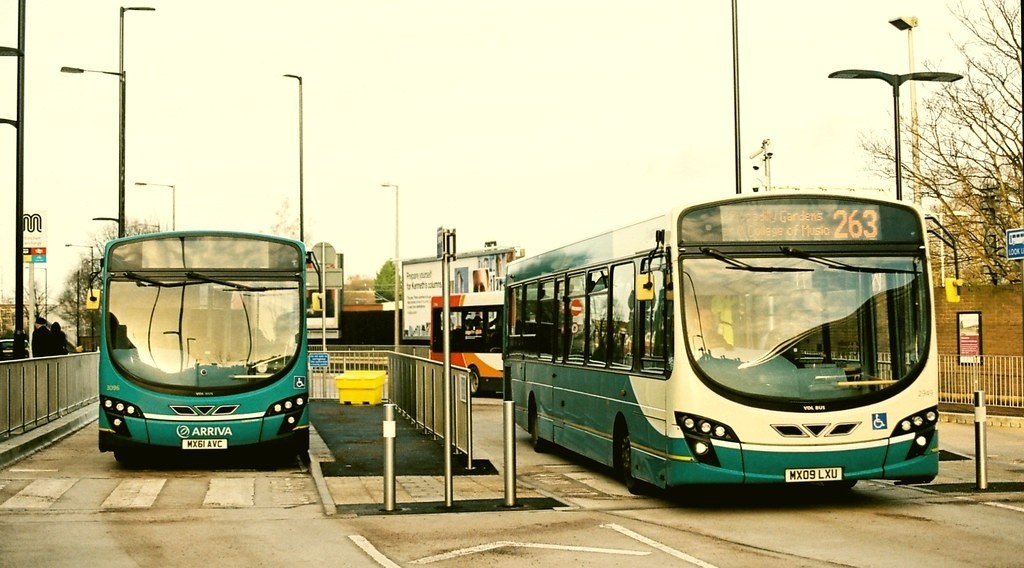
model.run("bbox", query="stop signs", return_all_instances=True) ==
[569,299,585,318]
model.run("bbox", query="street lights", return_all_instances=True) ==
[134,182,176,232]
[890,15,920,205]
[25,265,48,327]
[283,74,304,240]
[65,244,95,353]
[829,69,965,202]
[61,7,158,237]
[382,184,400,350]
[926,209,969,287]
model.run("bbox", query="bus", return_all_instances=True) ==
[428,286,562,399]
[500,188,964,500]
[84,230,324,466]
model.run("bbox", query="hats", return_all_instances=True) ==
[36,317,47,324]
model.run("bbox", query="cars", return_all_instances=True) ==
[0,339,30,361]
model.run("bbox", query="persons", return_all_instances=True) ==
[32,318,55,358]
[50,322,67,356]
